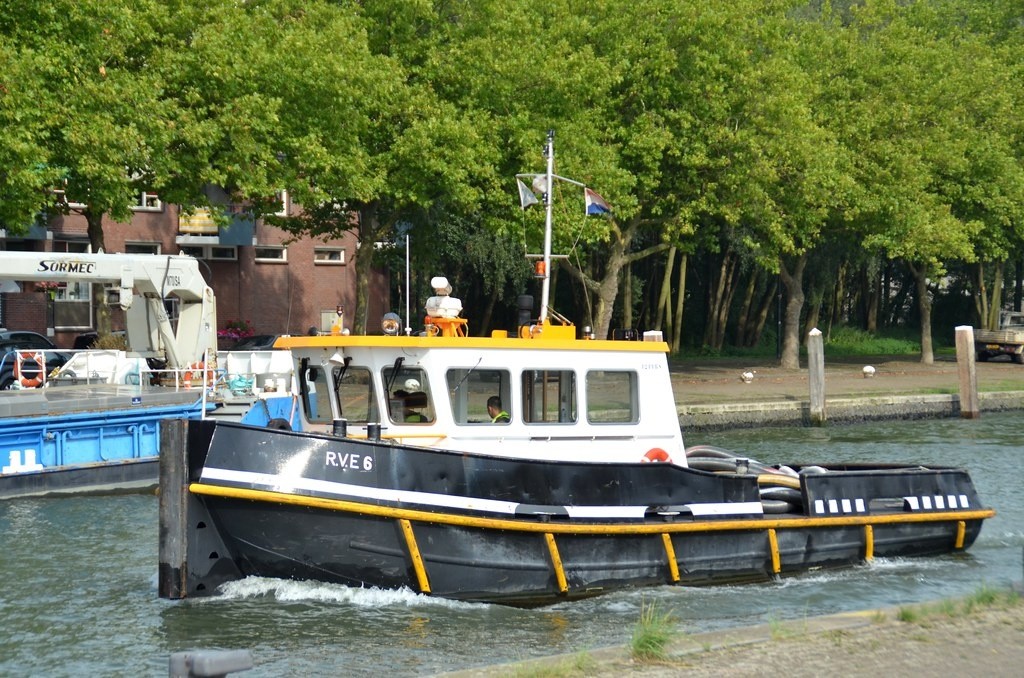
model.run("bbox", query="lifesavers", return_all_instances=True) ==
[13,353,42,387]
[641,448,673,463]
[183,362,213,388]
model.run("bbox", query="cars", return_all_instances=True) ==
[228,332,301,352]
[73,330,128,349]
[0,329,72,391]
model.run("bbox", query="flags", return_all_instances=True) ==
[585,188,612,216]
[516,178,539,207]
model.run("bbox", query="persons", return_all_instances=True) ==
[487,396,510,423]
[393,389,421,422]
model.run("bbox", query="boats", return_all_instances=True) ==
[0,348,317,501]
[186,138,996,613]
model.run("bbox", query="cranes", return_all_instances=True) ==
[0,251,218,387]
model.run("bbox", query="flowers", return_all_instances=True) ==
[36,282,61,293]
[218,318,256,340]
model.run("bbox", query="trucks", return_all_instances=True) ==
[973,312,1024,365]
